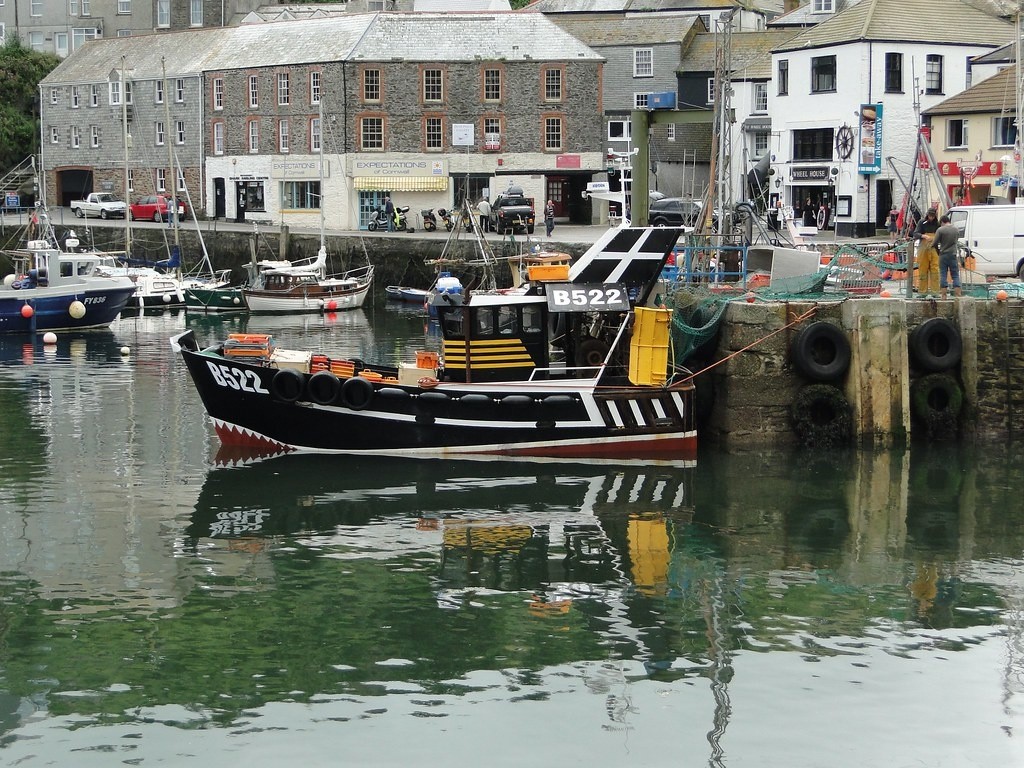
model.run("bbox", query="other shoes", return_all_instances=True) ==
[385,230,388,232]
[932,294,941,298]
[917,293,927,298]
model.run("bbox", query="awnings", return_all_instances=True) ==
[354,177,449,191]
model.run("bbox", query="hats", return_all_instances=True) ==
[927,207,936,214]
[385,197,390,201]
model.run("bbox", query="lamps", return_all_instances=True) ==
[854,111,859,117]
[827,176,836,187]
[775,176,783,189]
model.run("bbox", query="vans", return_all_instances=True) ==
[939,204,1024,283]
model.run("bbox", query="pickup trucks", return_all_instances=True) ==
[70,192,130,219]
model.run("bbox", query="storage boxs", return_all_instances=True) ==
[421,210,430,217]
[949,270,1024,299]
[223,334,439,385]
[742,245,821,292]
[527,265,570,281]
[840,279,881,293]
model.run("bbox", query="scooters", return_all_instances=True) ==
[459,204,474,233]
[368,204,409,232]
[419,207,437,232]
[438,208,458,232]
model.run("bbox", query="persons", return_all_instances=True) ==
[544,200,554,236]
[803,198,816,226]
[931,215,961,301]
[887,205,899,235]
[476,197,491,233]
[385,197,394,233]
[914,208,941,297]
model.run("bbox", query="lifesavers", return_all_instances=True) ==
[908,315,962,372]
[576,340,616,378]
[788,382,854,447]
[272,368,305,402]
[909,373,964,427]
[547,312,567,347]
[307,371,342,406]
[908,446,963,549]
[790,320,853,382]
[341,376,374,410]
[790,447,852,557]
[682,305,721,428]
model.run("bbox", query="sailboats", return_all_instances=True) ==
[106,54,375,317]
[817,55,959,286]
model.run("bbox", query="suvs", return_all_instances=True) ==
[648,189,741,232]
[489,193,535,234]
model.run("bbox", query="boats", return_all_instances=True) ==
[0,83,142,336]
[181,441,742,768]
[167,143,700,469]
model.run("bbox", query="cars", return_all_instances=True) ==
[129,194,188,223]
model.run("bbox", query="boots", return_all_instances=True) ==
[955,287,962,297]
[941,288,947,300]
